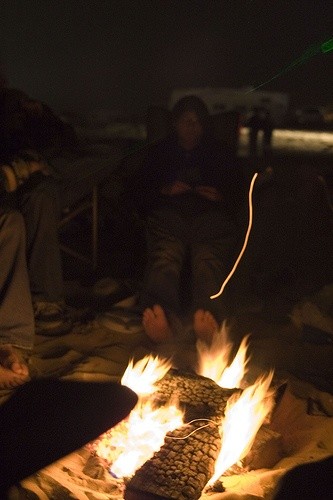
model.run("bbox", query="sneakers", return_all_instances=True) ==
[1,155,31,192]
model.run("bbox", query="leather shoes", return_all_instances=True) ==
[34,315,70,336]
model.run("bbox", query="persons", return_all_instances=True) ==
[0,67,81,344]
[261,110,275,151]
[248,108,261,157]
[1,192,39,354]
[113,92,244,346]
